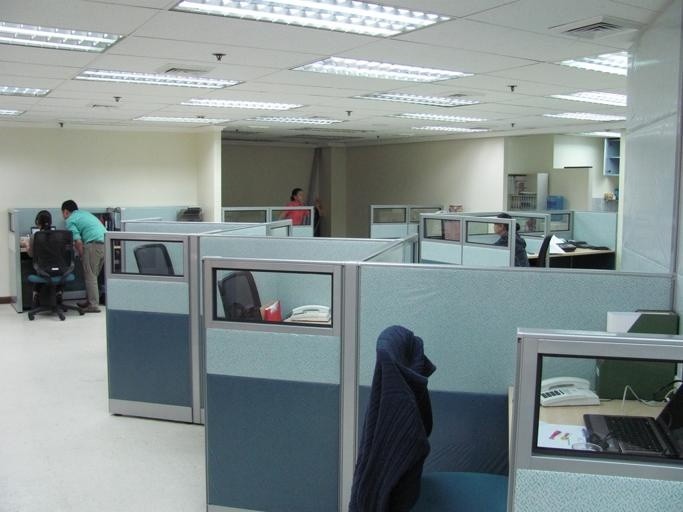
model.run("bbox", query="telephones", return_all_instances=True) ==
[292,304,332,322]
[540,376,601,407]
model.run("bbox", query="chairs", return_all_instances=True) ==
[372,317,509,511]
[127,242,175,276]
[24,229,85,320]
[534,233,556,267]
[215,269,264,323]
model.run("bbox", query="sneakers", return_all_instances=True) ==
[77,301,90,307]
[82,306,101,313]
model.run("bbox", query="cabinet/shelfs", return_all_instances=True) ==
[506,171,548,223]
[602,137,619,178]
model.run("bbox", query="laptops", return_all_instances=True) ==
[583,384,683,456]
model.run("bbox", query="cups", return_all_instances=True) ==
[571,442,602,452]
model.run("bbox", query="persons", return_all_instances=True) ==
[61,200,108,313]
[20,210,68,309]
[276,187,311,226]
[492,215,530,267]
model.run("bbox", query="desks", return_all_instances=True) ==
[520,240,616,269]
[281,311,329,324]
[8,238,121,314]
[505,387,668,458]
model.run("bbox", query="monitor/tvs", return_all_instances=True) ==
[30,226,56,234]
